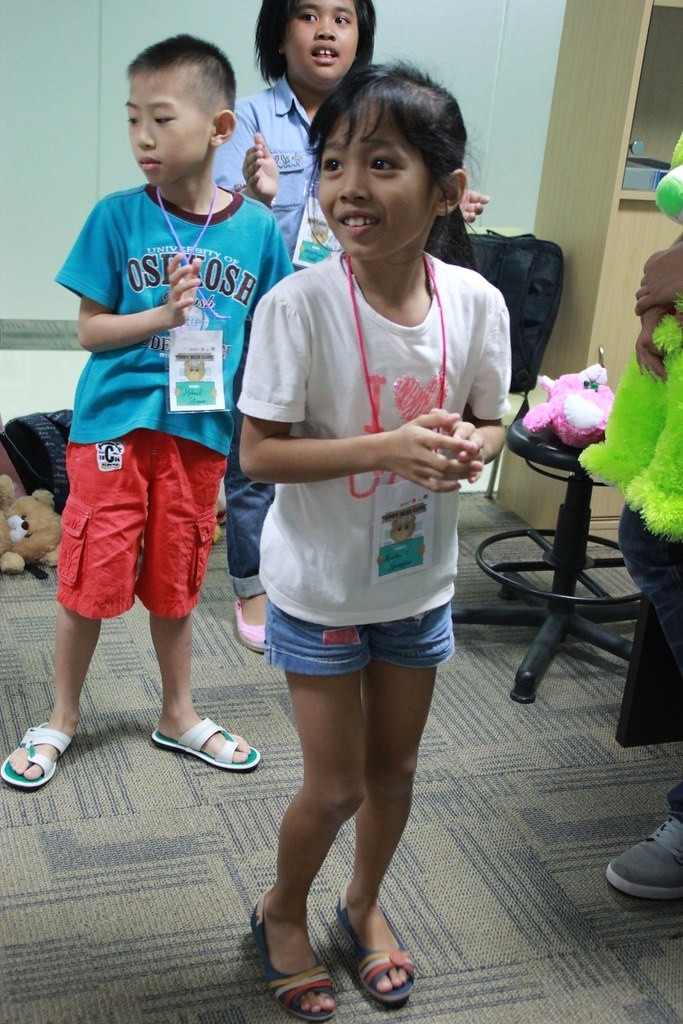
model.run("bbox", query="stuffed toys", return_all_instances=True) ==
[1,474,66,576]
[579,130,683,541]
[523,357,615,447]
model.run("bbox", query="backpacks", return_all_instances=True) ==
[1,406,91,515]
[466,229,566,391]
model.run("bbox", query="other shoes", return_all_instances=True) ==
[234,600,265,653]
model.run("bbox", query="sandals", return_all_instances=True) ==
[336,880,414,1004]
[251,893,336,1021]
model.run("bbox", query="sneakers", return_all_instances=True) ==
[606,817,683,899]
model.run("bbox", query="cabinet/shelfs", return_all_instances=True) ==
[495,1,683,548]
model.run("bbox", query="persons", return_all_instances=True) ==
[209,0,491,654]
[607,229,683,901]
[2,33,296,789]
[235,59,511,1023]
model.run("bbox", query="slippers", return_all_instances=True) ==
[151,717,260,772]
[1,722,71,789]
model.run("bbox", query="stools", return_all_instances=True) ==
[451,419,642,703]
